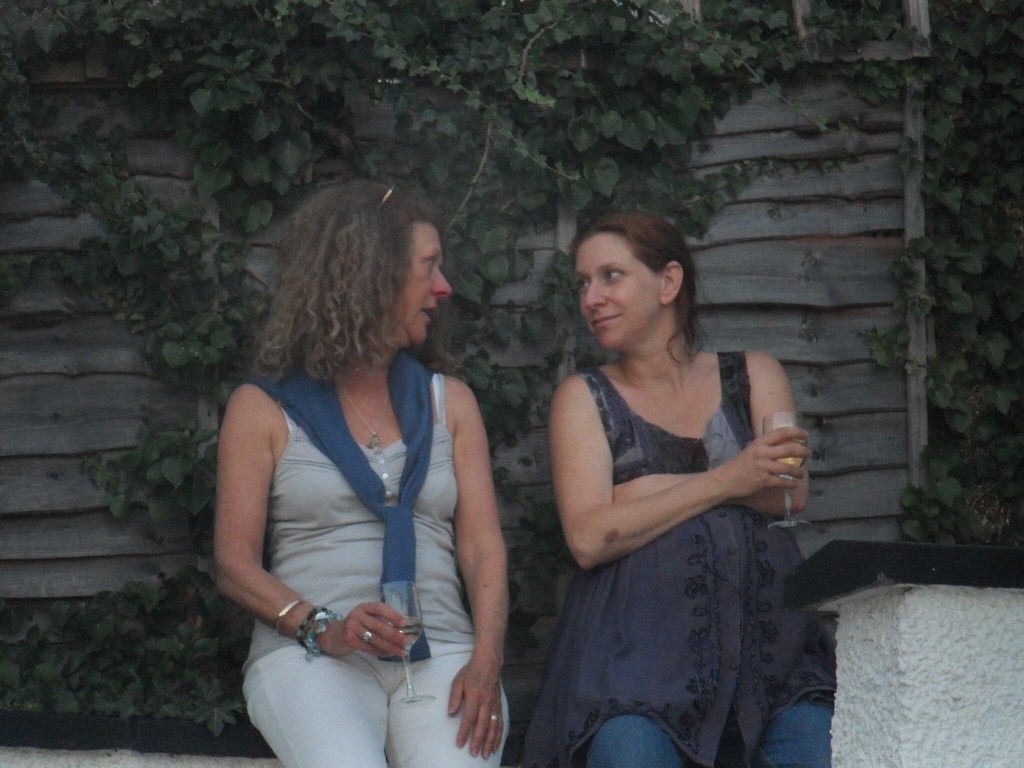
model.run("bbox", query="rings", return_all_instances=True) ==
[364,631,372,642]
[490,716,499,720]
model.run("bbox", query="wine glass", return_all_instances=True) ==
[763,410,814,530]
[378,580,436,711]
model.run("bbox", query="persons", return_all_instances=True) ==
[514,212,838,768]
[210,178,509,768]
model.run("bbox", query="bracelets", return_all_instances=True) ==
[297,603,343,661]
[277,598,304,634]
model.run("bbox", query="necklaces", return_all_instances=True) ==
[340,384,389,448]
